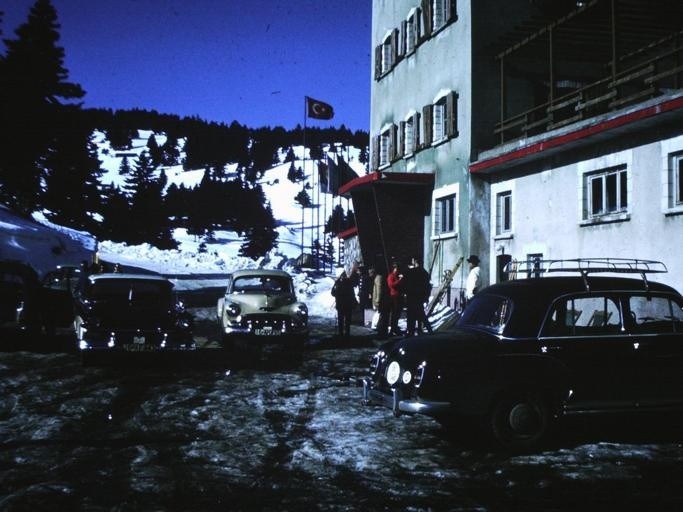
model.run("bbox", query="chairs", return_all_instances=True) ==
[563,307,611,327]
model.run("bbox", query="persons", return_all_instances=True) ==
[330,255,433,338]
[465,254,482,304]
[80,261,123,274]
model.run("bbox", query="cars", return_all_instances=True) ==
[0,257,57,355]
[71,272,197,370]
[359,255,682,456]
[217,267,310,345]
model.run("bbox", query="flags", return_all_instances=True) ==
[306,96,335,120]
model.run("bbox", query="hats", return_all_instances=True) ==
[466,255,480,264]
